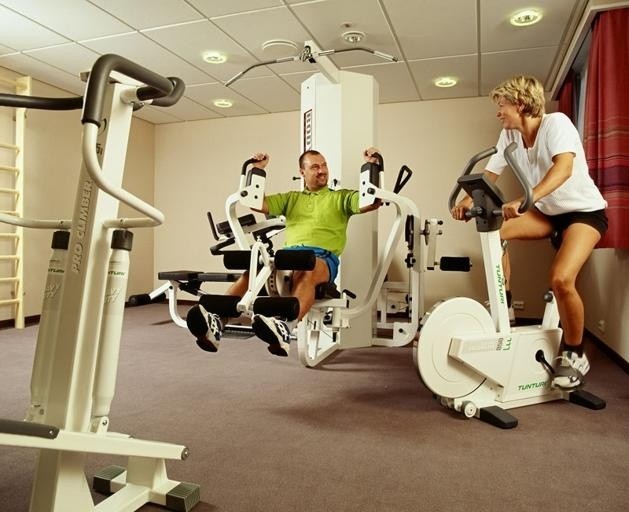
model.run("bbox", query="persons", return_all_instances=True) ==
[187,146,383,356]
[451,75,608,390]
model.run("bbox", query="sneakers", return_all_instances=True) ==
[485,303,516,326]
[184,302,225,353]
[551,350,591,391]
[250,312,292,357]
